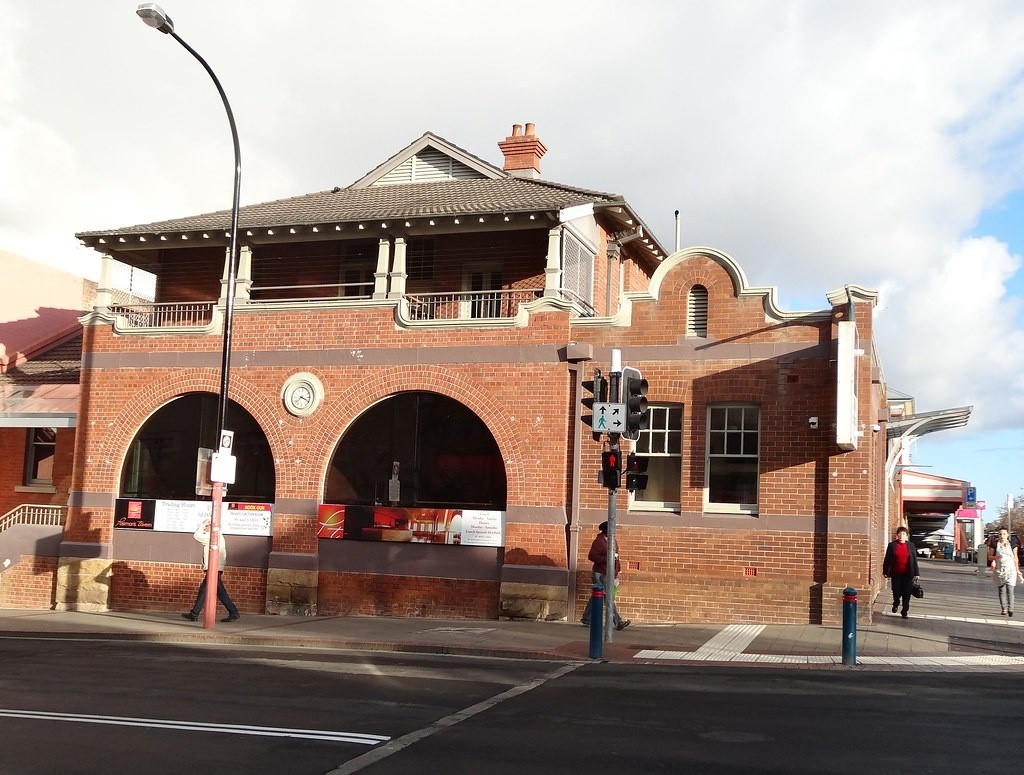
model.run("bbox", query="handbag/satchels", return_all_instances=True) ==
[604,578,619,603]
[911,579,923,598]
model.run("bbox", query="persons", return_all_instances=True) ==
[181,518,240,623]
[580,521,631,631]
[987,527,1023,617]
[883,527,920,619]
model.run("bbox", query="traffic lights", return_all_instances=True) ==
[601,451,622,488]
[620,366,649,442]
[580,376,608,442]
[626,452,649,491]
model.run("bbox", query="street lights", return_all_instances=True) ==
[135,1,243,631]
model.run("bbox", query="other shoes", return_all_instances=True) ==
[580,618,592,628]
[1008,610,1013,617]
[221,612,240,622]
[181,613,197,622]
[892,606,898,613]
[901,616,908,619]
[1002,607,1007,615]
[617,620,631,630]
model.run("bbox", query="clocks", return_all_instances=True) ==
[283,378,320,416]
